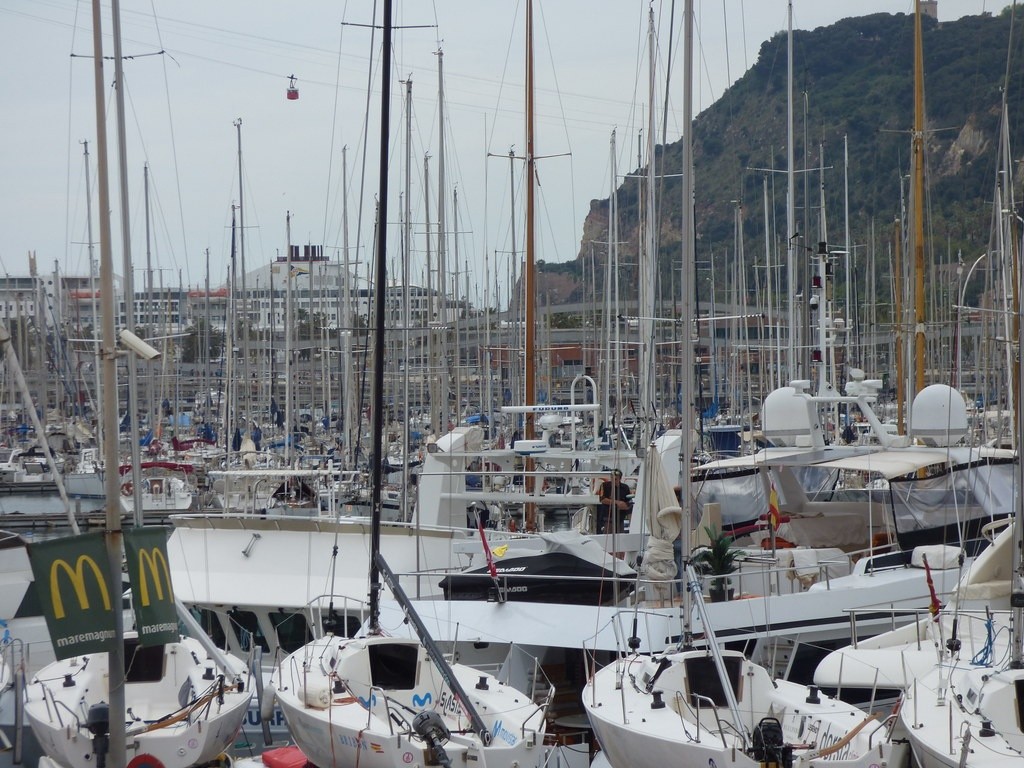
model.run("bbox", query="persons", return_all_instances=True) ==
[600,469,632,531]
[239,416,246,436]
[248,420,261,451]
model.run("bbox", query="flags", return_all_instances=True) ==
[924,559,941,621]
[24,530,121,663]
[123,525,180,647]
[271,396,284,430]
[770,482,781,531]
[473,512,499,580]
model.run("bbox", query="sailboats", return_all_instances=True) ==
[2,0,1022,768]
